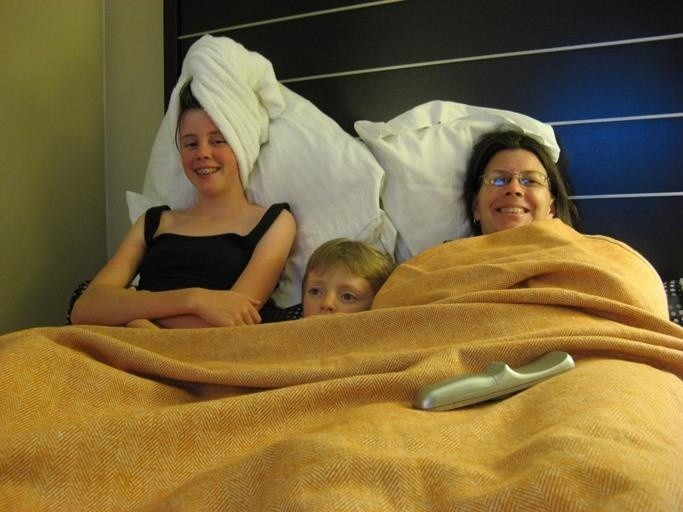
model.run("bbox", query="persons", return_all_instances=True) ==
[302,237,393,317]
[70,75,296,330]
[464,130,580,237]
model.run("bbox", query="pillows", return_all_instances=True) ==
[353,98,559,264]
[140,84,401,310]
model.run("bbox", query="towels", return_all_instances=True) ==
[168,35,285,193]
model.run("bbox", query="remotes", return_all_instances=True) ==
[412,352,575,412]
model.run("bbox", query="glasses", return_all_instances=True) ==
[479,171,551,191]
